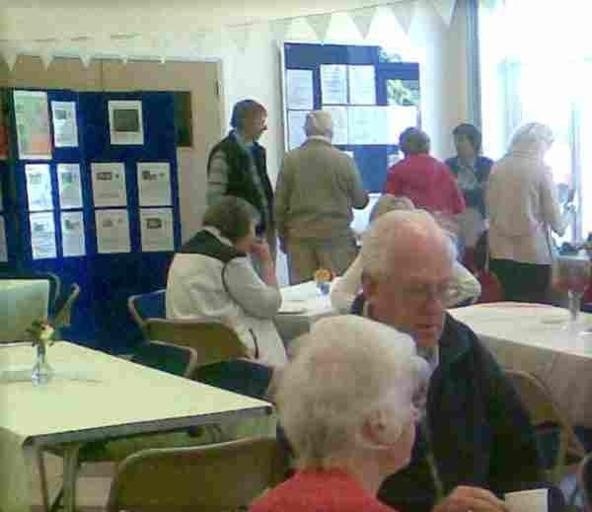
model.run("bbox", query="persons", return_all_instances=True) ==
[274,205,545,511]
[445,123,495,271]
[331,194,481,315]
[166,196,288,397]
[485,121,566,303]
[245,311,433,512]
[274,110,368,287]
[384,126,467,235]
[206,99,277,259]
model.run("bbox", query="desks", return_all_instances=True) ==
[0,336,272,512]
[444,300,592,431]
[270,268,361,333]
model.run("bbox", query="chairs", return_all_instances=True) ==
[125,288,591,512]
[1,262,80,343]
